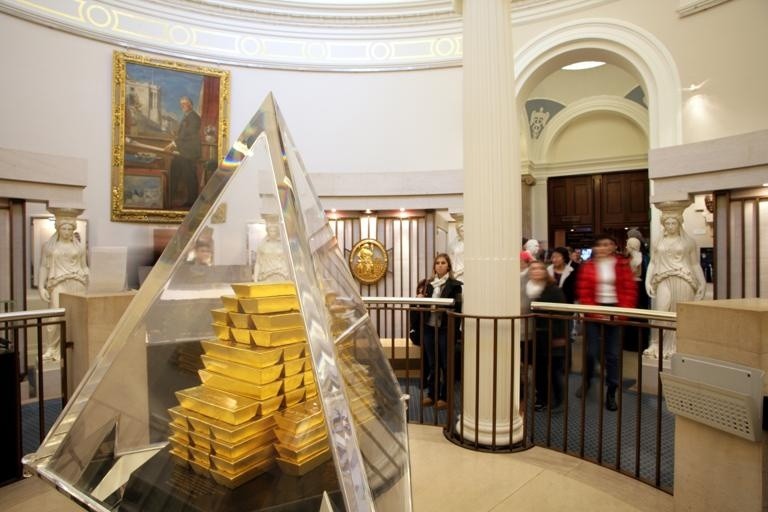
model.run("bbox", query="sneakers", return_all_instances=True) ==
[433,400,446,409]
[606,393,617,411]
[423,397,432,406]
[533,403,563,414]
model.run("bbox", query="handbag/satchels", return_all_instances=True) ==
[410,304,420,346]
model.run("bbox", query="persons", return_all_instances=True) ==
[642,207,709,358]
[447,223,463,282]
[356,243,377,278]
[414,253,463,409]
[250,222,289,283]
[519,227,650,412]
[161,95,201,211]
[181,238,211,266]
[37,215,91,362]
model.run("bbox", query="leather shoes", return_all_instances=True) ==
[577,381,590,398]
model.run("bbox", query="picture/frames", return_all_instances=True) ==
[110,49,232,225]
